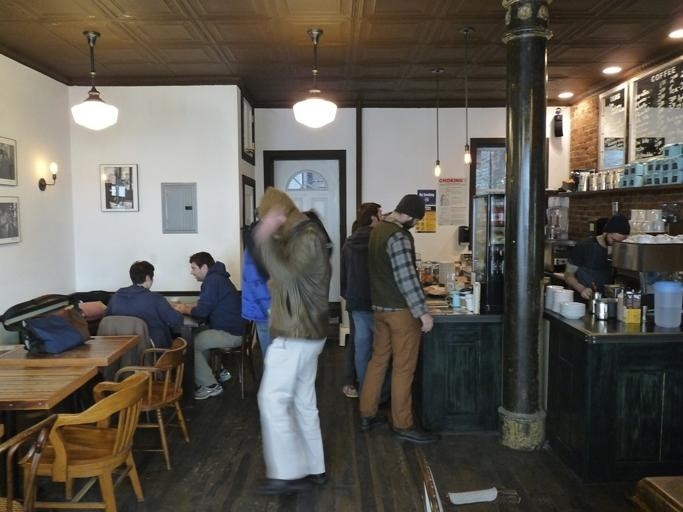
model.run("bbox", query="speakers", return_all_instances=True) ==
[554,114,564,138]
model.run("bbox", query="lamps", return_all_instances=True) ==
[462,26,473,167]
[69,29,120,130]
[293,27,338,128]
[432,66,444,178]
[39,162,58,191]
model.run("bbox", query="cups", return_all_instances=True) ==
[464,294,474,310]
[628,208,665,232]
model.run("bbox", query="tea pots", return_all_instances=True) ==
[445,293,461,308]
[588,290,602,314]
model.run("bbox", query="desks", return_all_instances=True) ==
[180,307,206,352]
[629,475,683,512]
[1,366,98,411]
[3,335,141,367]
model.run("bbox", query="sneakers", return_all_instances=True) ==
[218,368,232,381]
[343,383,360,399]
[194,384,223,399]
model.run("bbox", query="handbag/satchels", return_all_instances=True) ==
[22,304,90,354]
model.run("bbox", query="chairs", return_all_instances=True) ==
[112,337,191,471]
[96,316,158,383]
[20,371,152,510]
[207,318,256,401]
[0,414,59,511]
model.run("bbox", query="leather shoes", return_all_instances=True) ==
[257,471,327,494]
[361,412,389,430]
[396,425,441,443]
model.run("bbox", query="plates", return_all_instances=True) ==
[545,285,586,319]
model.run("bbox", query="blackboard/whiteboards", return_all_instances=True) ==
[628,55,683,164]
[597,81,628,171]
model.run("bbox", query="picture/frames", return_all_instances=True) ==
[0,195,21,245]
[0,136,19,186]
[100,164,139,212]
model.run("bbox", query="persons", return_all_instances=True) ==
[105,260,184,416]
[340,202,391,405]
[177,252,251,400]
[359,193,442,444]
[563,215,631,300]
[240,207,276,356]
[251,186,333,496]
[342,220,359,398]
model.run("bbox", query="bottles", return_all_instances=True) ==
[490,197,504,276]
[662,203,680,223]
[590,280,598,290]
[617,286,640,322]
[569,167,620,192]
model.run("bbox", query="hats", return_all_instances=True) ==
[604,214,630,235]
[395,194,425,220]
[256,188,294,220]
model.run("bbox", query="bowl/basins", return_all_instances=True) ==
[596,298,617,319]
